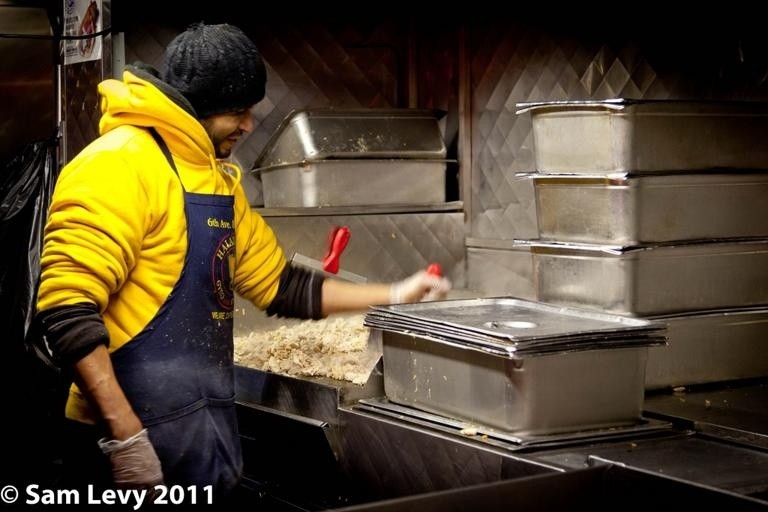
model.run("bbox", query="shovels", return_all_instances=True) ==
[351,263,442,385]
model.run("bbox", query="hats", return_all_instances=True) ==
[165,23,266,118]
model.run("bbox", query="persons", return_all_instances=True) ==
[34,23,452,512]
